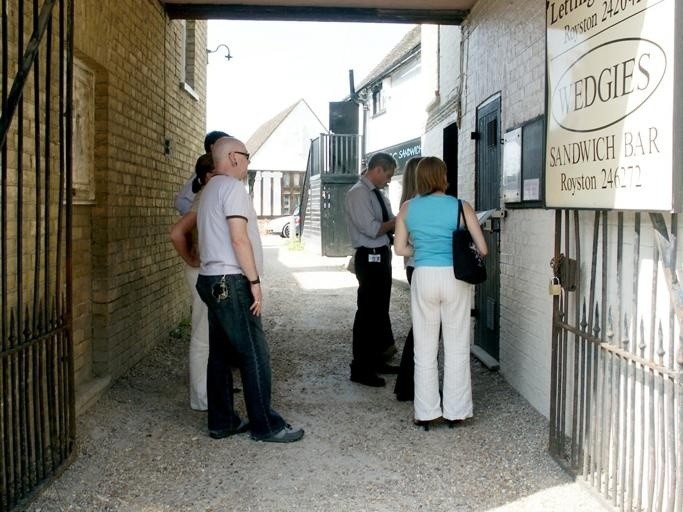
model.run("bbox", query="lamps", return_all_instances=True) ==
[207,43,232,62]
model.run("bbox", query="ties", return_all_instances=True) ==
[373,189,394,245]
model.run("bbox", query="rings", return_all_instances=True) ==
[257,302,260,305]
[254,301,258,304]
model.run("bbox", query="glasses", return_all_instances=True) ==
[229,151,249,160]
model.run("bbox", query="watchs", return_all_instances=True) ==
[248,274,261,286]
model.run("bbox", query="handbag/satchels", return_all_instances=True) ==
[452,199,488,285]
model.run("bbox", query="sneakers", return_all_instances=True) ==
[350,351,460,431]
[190,386,304,443]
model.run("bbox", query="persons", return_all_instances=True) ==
[172,130,235,216]
[392,156,444,402]
[169,136,305,443]
[345,150,401,388]
[357,166,399,363]
[181,150,243,415]
[393,154,489,430]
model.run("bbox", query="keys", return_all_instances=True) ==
[216,274,229,303]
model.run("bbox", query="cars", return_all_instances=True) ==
[262,205,300,239]
